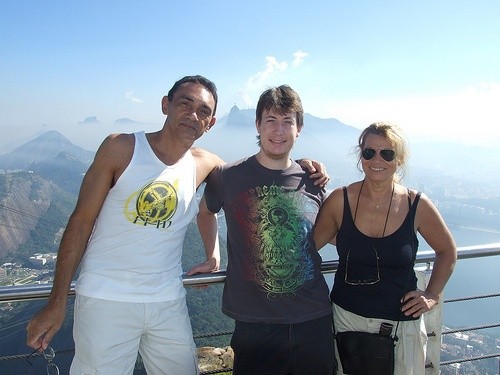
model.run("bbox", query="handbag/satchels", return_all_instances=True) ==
[333,330,399,375]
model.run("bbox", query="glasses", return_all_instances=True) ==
[26,342,60,375]
[362,148,396,163]
[344,245,380,286]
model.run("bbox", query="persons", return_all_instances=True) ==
[26,75,332,375]
[313,121,457,375]
[184,84,339,375]
